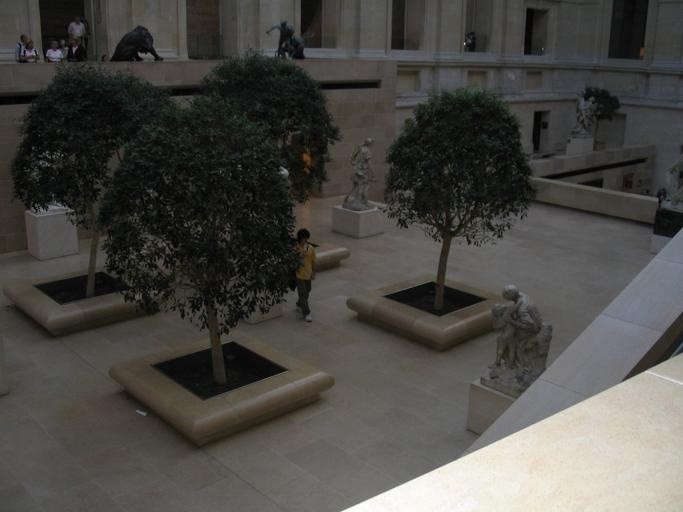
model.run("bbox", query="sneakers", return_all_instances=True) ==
[296,305,302,313]
[305,314,312,322]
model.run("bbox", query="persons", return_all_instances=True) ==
[14,17,88,63]
[264,19,294,56]
[277,38,307,59]
[571,88,596,134]
[343,136,375,206]
[492,302,515,366]
[464,32,470,51]
[465,32,476,52]
[501,284,542,340]
[294,228,316,321]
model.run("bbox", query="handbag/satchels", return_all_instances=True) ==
[290,271,297,292]
